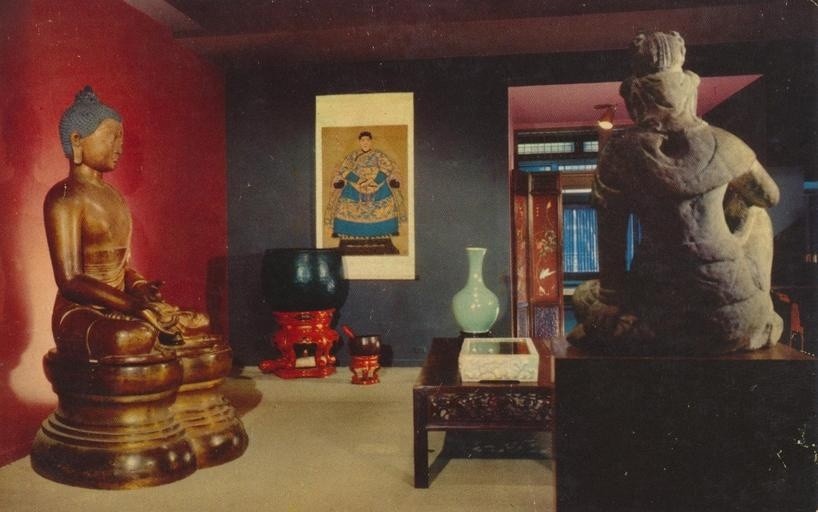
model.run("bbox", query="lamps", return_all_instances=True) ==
[596,104,618,130]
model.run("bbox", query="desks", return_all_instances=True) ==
[413,337,554,490]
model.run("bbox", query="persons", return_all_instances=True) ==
[43,84,211,360]
[570,31,785,354]
[325,131,407,238]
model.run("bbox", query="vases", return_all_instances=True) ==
[451,247,500,336]
